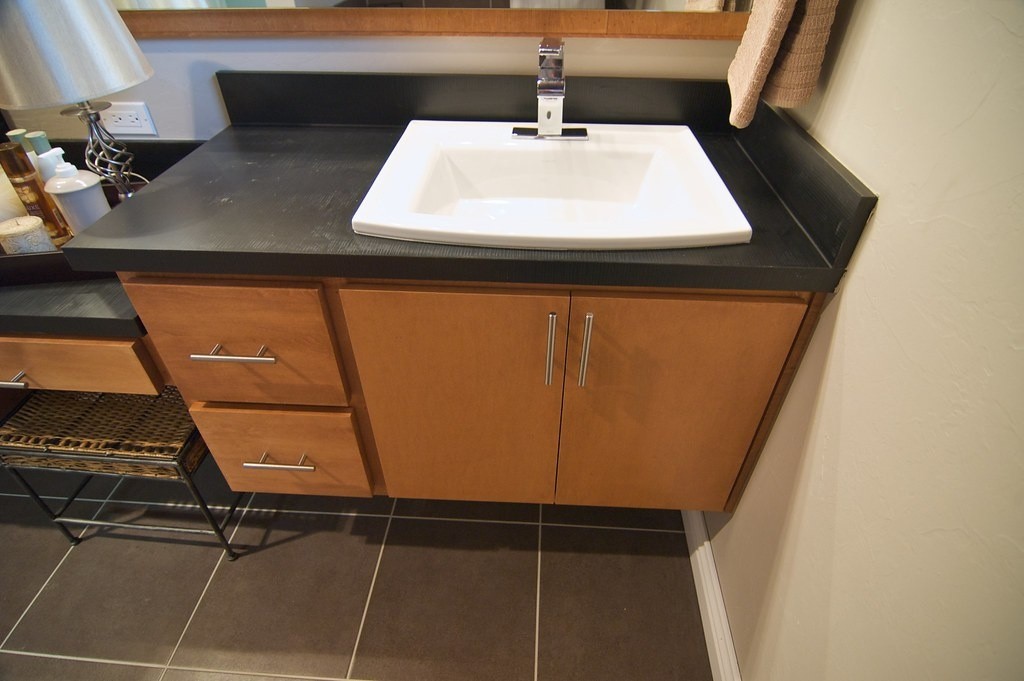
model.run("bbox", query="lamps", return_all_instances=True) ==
[0,1,154,201]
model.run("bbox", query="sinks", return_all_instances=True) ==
[351,116,754,252]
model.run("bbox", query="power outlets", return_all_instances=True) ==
[99,101,157,136]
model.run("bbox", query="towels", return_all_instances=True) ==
[725,0,838,131]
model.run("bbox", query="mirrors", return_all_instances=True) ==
[108,0,754,15]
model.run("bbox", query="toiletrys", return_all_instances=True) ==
[7,128,41,172]
[36,144,114,238]
[0,141,70,239]
[25,130,63,182]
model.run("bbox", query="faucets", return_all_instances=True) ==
[535,32,568,138]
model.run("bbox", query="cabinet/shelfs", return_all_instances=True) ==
[0,313,239,563]
[338,284,826,512]
[115,271,376,499]
